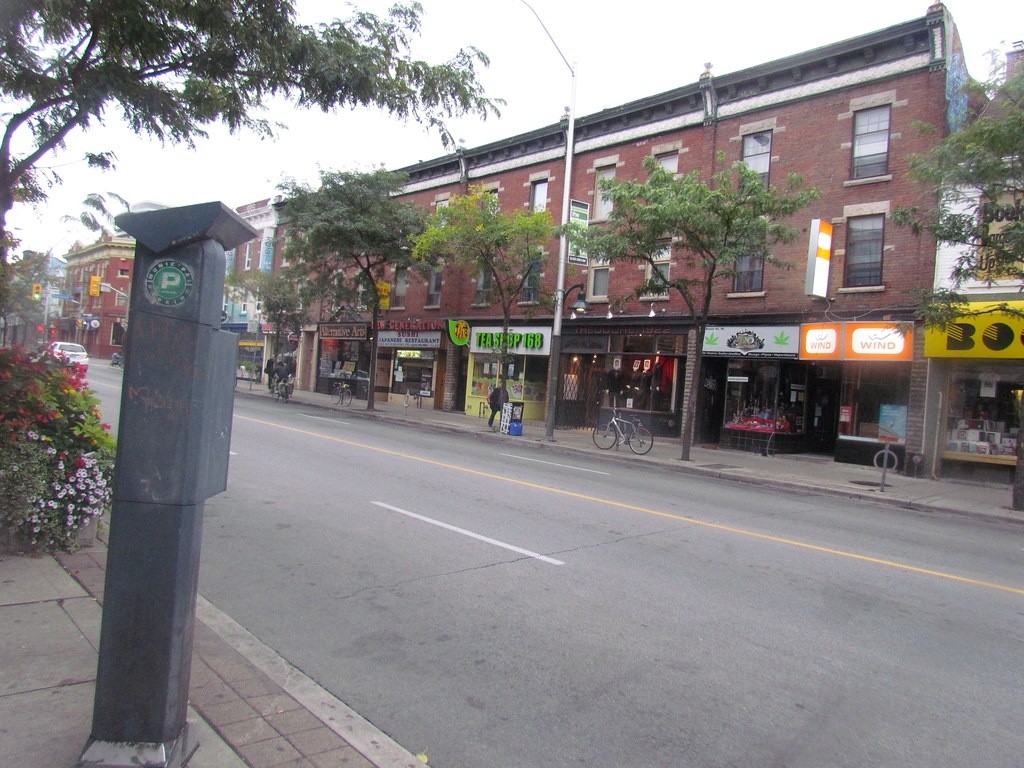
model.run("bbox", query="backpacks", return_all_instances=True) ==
[487,393,491,404]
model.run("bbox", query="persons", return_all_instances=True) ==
[889,421,896,429]
[267,354,293,394]
[634,361,639,367]
[270,363,289,394]
[418,382,432,391]
[488,382,509,427]
[645,361,649,367]
[605,349,665,410]
[628,400,631,406]
[513,407,521,419]
[615,360,619,366]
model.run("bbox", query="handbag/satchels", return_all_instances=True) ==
[264,368,268,373]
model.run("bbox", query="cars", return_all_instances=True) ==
[112,351,123,365]
[42,342,88,373]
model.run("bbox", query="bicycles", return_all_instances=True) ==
[270,376,292,403]
[331,382,352,407]
[591,405,654,456]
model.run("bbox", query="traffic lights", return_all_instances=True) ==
[38,325,43,331]
[51,328,55,336]
[31,283,41,301]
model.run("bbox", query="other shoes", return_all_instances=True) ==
[488,422,493,427]
